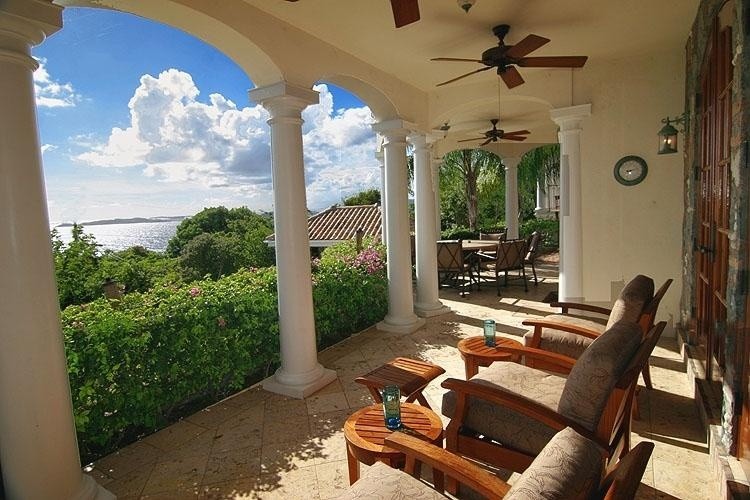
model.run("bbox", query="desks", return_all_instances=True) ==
[343,401,445,495]
[457,336,524,383]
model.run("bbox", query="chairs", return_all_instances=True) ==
[441,321,667,497]
[334,426,655,500]
[436,228,540,298]
[522,274,673,421]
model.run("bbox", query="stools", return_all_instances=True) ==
[354,356,445,412]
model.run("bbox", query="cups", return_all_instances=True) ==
[483,320,496,346]
[383,385,401,428]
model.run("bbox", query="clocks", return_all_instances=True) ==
[614,155,648,187]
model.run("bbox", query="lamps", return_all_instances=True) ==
[657,110,690,155]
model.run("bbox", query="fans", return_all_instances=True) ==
[458,120,531,146]
[283,0,420,29]
[430,25,588,90]
